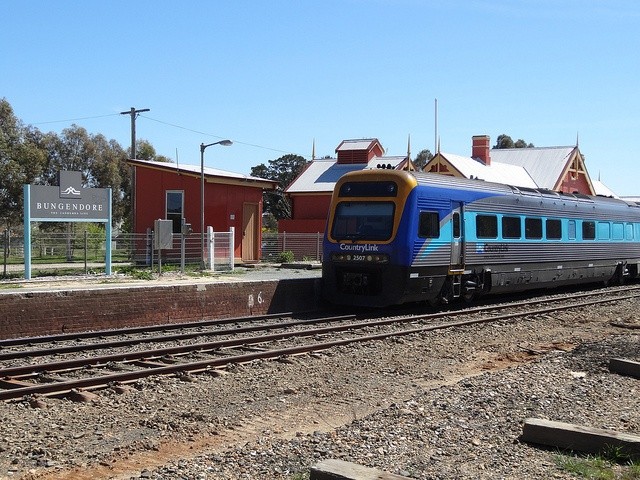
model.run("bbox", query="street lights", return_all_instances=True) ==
[200,140,232,270]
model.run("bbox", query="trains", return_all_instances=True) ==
[322,169,640,313]
